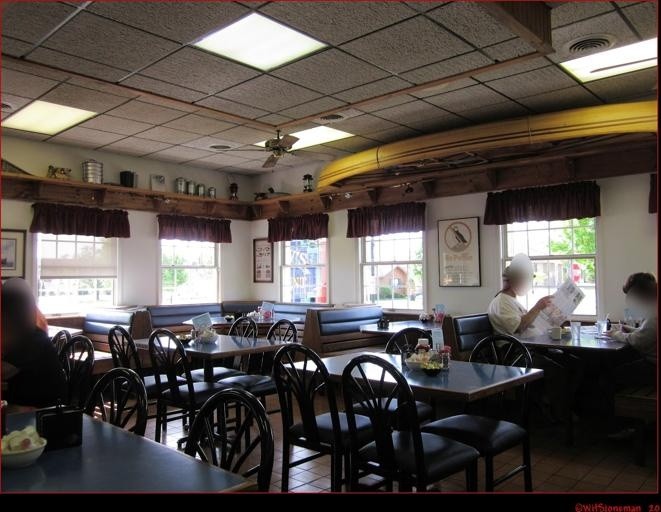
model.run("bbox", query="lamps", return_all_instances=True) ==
[318,173,442,208]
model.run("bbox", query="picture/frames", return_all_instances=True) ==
[253,238,274,283]
[1,226,27,280]
[438,216,483,289]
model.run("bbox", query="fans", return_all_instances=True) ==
[217,130,336,169]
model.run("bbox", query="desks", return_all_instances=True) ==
[283,350,546,492]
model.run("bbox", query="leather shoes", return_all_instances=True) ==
[533,397,561,426]
[606,428,639,444]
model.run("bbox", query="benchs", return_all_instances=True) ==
[295,291,659,462]
[0,295,297,492]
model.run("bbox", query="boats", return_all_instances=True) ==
[315,97,659,192]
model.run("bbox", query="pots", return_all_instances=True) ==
[377,320,391,328]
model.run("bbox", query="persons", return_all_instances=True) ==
[575,272,658,442]
[1,280,67,405]
[487,263,585,452]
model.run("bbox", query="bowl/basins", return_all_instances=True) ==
[407,360,423,372]
[225,318,233,321]
[423,367,442,377]
[1,438,47,470]
[177,338,193,344]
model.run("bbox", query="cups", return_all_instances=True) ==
[548,326,563,340]
[595,319,610,338]
[612,323,623,333]
[571,322,582,340]
[571,339,581,346]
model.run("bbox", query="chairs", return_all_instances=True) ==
[274,342,379,493]
[422,334,537,491]
[343,354,482,492]
[351,328,436,486]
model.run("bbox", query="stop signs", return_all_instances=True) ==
[567,263,581,282]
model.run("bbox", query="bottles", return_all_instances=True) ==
[415,338,432,355]
